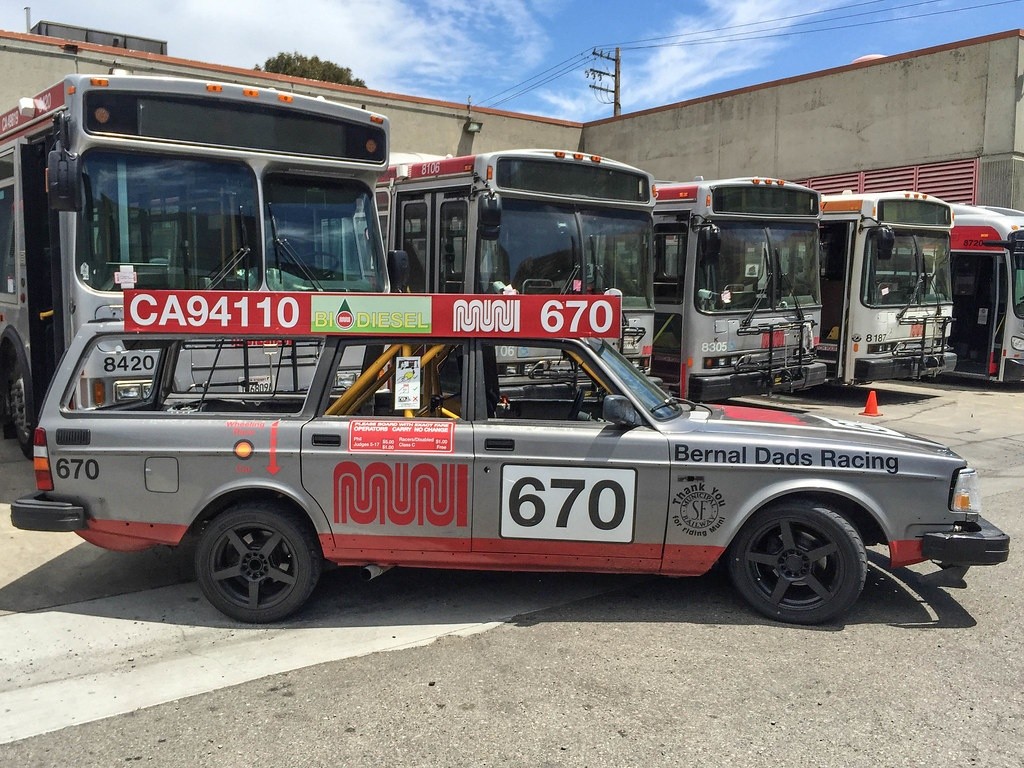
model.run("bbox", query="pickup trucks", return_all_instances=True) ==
[9,288,1009,626]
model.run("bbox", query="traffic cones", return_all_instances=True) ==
[859,390,883,416]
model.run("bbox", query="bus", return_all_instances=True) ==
[0,63,394,463]
[648,175,829,406]
[950,201,1024,387]
[820,189,957,392]
[373,147,665,418]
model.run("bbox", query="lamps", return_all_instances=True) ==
[464,119,483,134]
[108,68,136,76]
